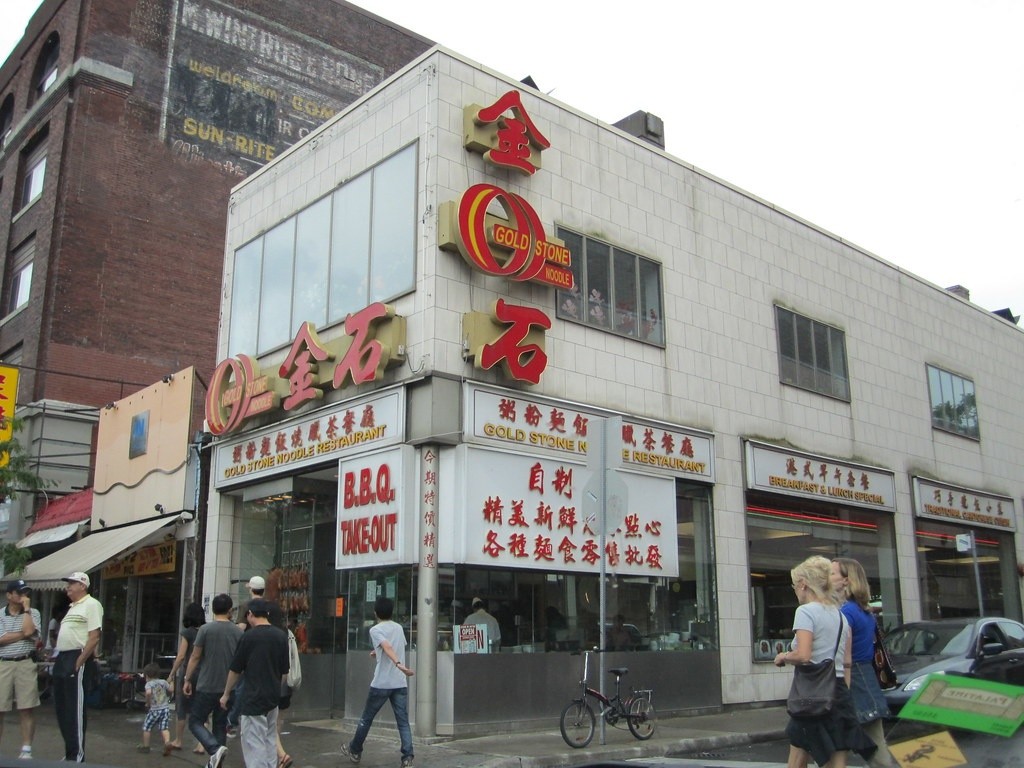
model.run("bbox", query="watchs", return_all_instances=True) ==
[184,675,190,681]
[24,610,32,614]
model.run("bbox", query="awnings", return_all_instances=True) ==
[0,515,181,591]
[14,518,91,548]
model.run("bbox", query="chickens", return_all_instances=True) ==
[267,563,309,655]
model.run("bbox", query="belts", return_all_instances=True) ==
[0,654,30,661]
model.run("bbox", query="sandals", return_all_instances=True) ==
[278,753,293,768]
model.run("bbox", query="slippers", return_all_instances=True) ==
[171,744,181,750]
[193,749,204,754]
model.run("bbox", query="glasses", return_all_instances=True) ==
[14,590,28,596]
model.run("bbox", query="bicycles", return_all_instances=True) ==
[559,647,654,746]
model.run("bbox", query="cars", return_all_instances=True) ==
[869,612,1023,743]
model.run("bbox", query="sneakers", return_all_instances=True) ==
[401,759,414,768]
[205,746,228,768]
[19,751,33,759]
[341,744,361,763]
[138,744,150,753]
[163,742,173,755]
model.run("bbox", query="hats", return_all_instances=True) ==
[472,597,482,605]
[451,600,463,608]
[246,576,265,589]
[7,580,33,592]
[61,572,90,588]
[244,598,269,616]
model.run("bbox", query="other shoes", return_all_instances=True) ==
[227,729,237,738]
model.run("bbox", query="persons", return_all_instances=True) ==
[219,598,288,768]
[44,607,66,650]
[236,575,266,631]
[608,615,631,652]
[462,598,501,653]
[183,593,244,768]
[49,572,104,761]
[0,579,43,759]
[774,556,878,768]
[831,558,898,768]
[340,597,415,768]
[245,600,302,768]
[137,662,172,756]
[165,602,206,752]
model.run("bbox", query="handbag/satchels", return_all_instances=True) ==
[286,628,302,689]
[873,621,897,689]
[787,659,836,717]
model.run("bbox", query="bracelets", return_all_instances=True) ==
[395,661,400,666]
[784,651,790,661]
